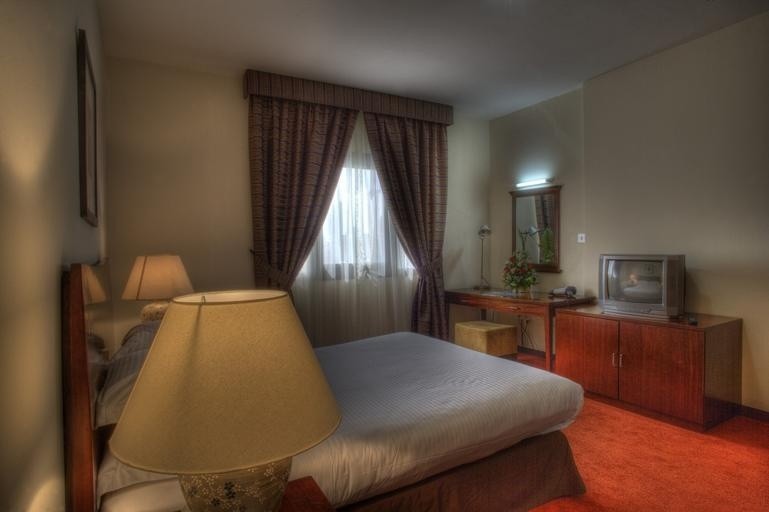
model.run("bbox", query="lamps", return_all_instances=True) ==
[109,288,342,512]
[119,254,194,324]
[473,225,491,291]
[518,225,537,255]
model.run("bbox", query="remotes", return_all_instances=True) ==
[687,317,698,325]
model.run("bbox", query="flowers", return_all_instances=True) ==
[503,252,539,291]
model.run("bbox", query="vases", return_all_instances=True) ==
[512,286,533,301]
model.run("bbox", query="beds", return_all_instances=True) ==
[62,262,587,512]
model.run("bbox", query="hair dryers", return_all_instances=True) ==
[548,285,576,298]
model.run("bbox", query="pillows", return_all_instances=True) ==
[95,319,177,512]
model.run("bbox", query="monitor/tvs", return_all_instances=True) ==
[599,253,686,321]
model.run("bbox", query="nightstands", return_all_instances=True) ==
[280,474,336,511]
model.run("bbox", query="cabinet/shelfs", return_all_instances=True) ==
[553,299,743,429]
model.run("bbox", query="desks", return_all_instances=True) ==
[444,286,597,373]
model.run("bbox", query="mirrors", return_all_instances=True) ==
[508,184,563,273]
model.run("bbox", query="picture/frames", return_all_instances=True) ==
[76,28,103,228]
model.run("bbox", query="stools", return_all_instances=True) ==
[454,320,519,362]
